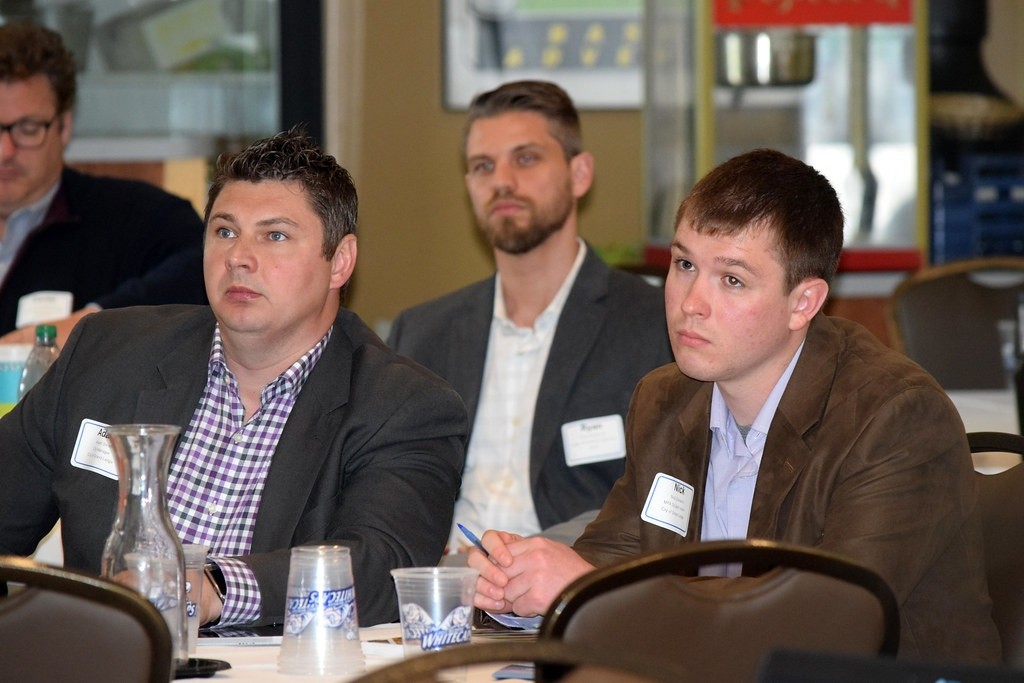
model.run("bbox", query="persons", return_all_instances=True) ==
[461,148,1002,683]
[382,80,676,555]
[0,18,208,346]
[1,123,471,637]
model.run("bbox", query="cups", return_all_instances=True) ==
[391,567,483,659]
[181,543,211,655]
[277,546,366,678]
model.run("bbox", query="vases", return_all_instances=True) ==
[98,424,188,669]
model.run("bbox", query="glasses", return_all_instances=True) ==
[0,108,62,149]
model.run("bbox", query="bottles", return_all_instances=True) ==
[101,423,187,680]
[18,325,61,403]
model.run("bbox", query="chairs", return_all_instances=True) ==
[883,256,1024,438]
[0,553,175,683]
[965,431,1024,683]
[344,538,903,683]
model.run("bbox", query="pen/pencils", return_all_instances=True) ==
[457,523,489,556]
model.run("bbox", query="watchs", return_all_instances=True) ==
[205,562,227,602]
